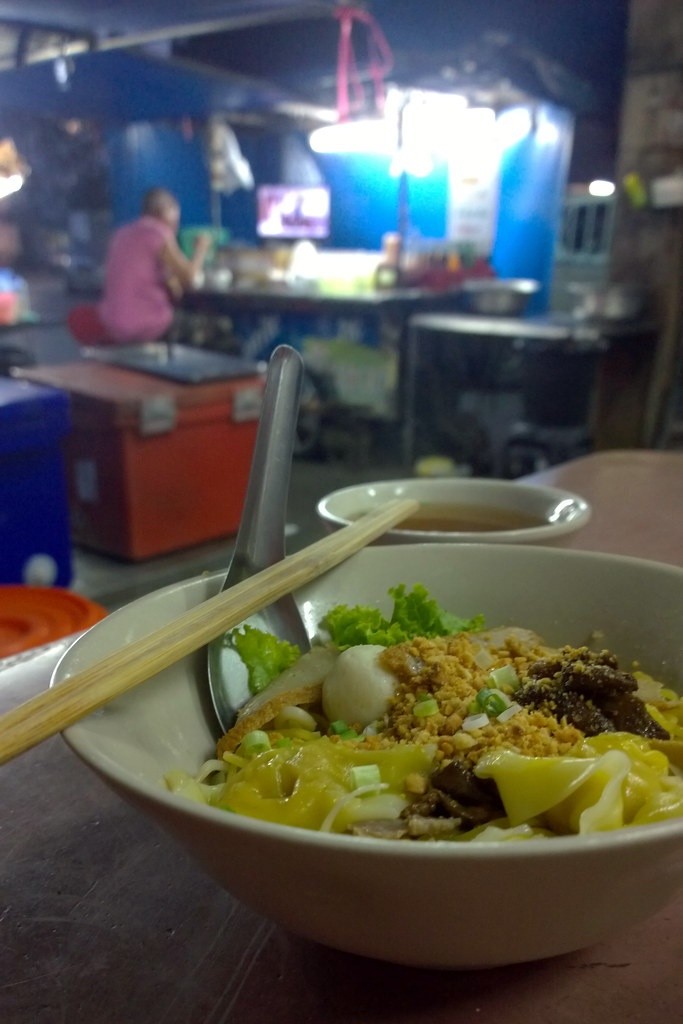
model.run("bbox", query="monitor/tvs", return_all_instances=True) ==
[255,185,331,238]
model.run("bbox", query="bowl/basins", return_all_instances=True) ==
[316,477,592,546]
[460,277,542,315]
[46,543,683,967]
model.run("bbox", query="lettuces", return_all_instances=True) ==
[225,582,487,697]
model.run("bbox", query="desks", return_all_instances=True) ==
[403,308,662,483]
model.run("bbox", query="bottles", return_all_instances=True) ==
[370,233,402,287]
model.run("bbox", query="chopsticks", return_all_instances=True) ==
[0,498,425,770]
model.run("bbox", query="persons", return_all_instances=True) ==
[95,188,245,357]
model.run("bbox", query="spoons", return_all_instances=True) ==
[204,343,313,742]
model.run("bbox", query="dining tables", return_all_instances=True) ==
[1,446,683,1024]
